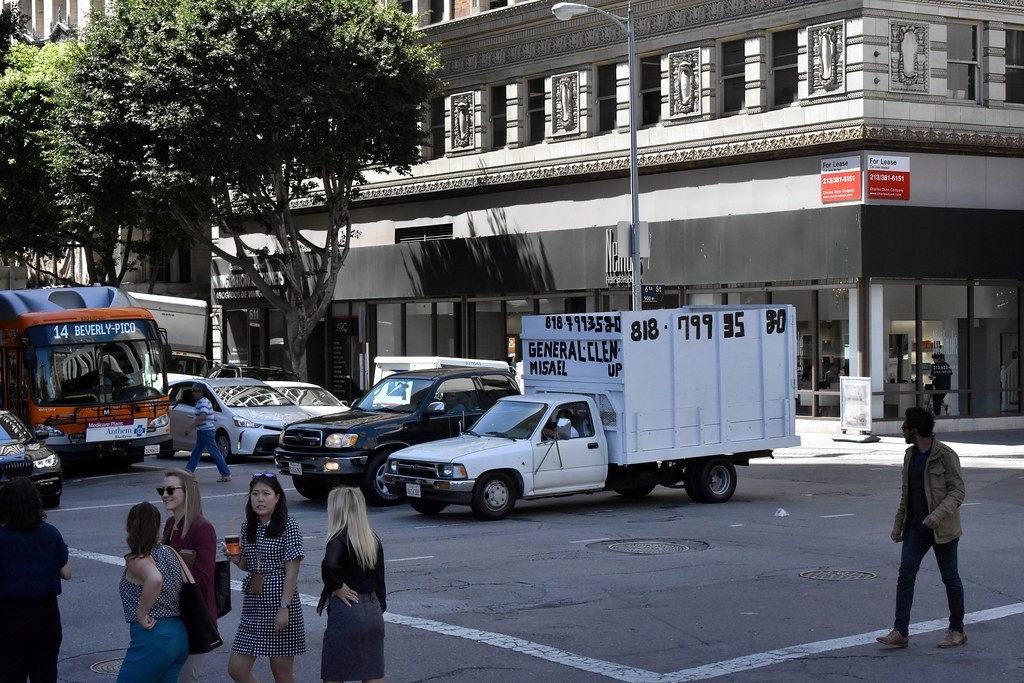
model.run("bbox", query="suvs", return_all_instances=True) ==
[206,362,301,383]
[272,367,521,507]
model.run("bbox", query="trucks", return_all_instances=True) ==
[0,283,173,472]
[385,303,802,522]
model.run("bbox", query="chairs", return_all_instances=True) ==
[571,415,584,438]
[800,383,840,417]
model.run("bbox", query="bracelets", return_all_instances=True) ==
[233,557,241,564]
[279,603,290,609]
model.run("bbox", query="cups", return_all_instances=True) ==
[224,535,241,557]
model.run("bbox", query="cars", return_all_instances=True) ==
[0,408,66,509]
[261,380,353,417]
[154,376,315,465]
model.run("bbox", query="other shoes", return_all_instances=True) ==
[217,475,232,482]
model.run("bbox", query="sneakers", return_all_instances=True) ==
[876,628,909,647]
[937,629,968,648]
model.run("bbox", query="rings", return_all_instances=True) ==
[347,595,350,598]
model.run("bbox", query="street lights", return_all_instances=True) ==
[550,0,643,310]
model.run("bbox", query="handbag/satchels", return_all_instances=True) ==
[168,548,223,654]
[214,561,232,619]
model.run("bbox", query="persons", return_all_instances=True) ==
[876,406,968,648]
[117,501,189,683]
[184,383,232,482]
[316,488,387,683]
[930,353,953,416]
[0,477,71,683]
[156,468,217,683]
[221,471,306,683]
[825,360,849,381]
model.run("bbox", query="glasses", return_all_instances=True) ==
[902,427,911,430]
[157,487,183,496]
[253,472,278,479]
